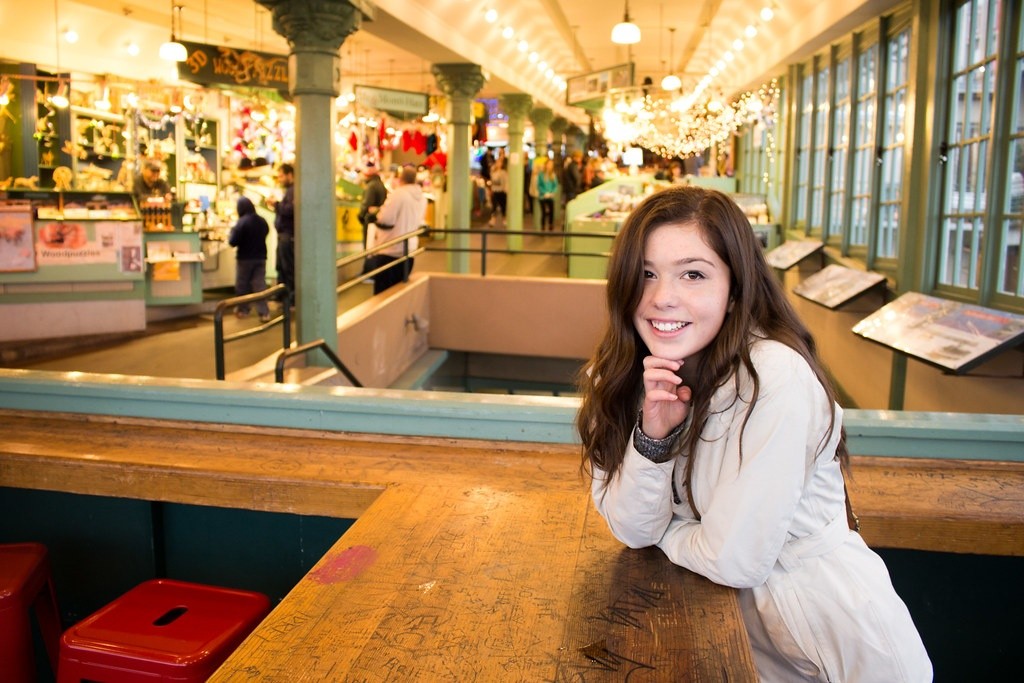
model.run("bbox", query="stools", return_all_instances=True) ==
[56,577,269,683]
[0,542,64,683]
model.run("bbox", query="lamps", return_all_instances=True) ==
[159,0,188,62]
[611,0,640,44]
[662,27,682,90]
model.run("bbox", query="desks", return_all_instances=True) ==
[1,408,1024,683]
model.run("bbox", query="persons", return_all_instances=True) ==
[132,163,177,205]
[478,146,509,228]
[523,150,534,216]
[559,156,573,198]
[530,145,548,218]
[229,197,269,323]
[238,158,253,171]
[572,186,933,682]
[369,165,427,296]
[671,160,682,177]
[254,157,270,167]
[358,161,388,273]
[571,149,586,194]
[267,162,296,314]
[116,137,148,187]
[537,159,558,231]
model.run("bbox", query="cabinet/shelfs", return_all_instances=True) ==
[0,63,221,227]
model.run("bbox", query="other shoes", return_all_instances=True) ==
[234,308,245,317]
[261,314,270,322]
